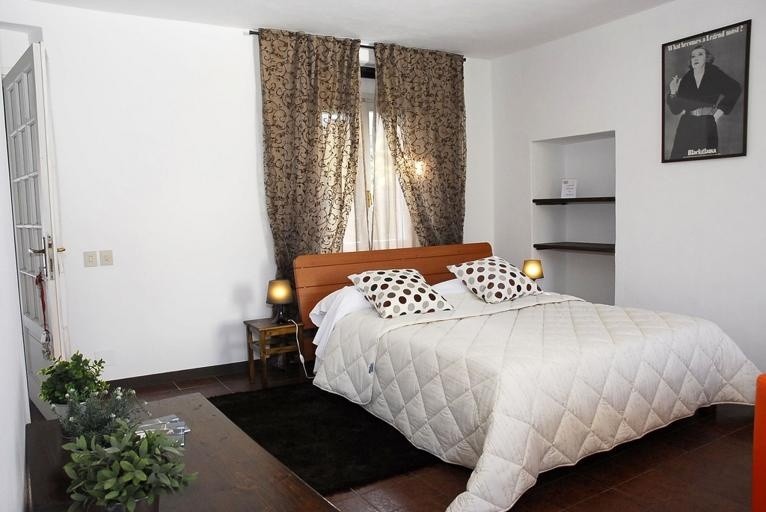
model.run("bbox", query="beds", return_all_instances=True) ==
[292,242,718,490]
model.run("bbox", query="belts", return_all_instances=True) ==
[691,107,713,116]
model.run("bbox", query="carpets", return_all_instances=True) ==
[207,381,439,496]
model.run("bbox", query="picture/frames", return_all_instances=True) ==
[662,20,751,162]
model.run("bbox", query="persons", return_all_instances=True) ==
[667,45,743,159]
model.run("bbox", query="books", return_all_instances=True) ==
[131,413,193,448]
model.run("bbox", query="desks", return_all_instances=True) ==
[244,318,305,388]
[26,393,341,512]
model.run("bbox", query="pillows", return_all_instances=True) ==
[444,256,545,304]
[346,268,455,319]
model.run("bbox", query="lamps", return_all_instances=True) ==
[522,260,545,282]
[266,280,294,324]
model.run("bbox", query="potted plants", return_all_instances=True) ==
[36,350,200,512]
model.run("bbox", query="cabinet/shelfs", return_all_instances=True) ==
[532,197,615,252]
